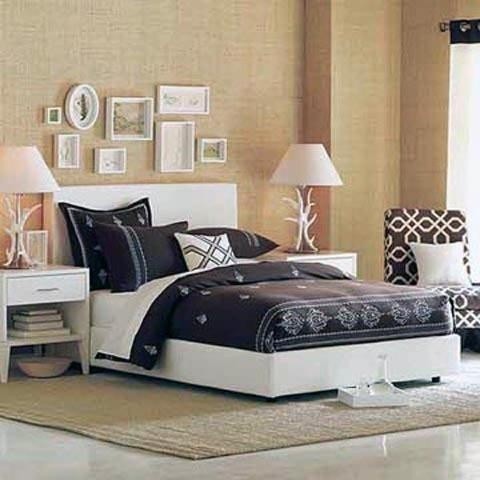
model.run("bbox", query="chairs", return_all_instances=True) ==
[383,206,480,352]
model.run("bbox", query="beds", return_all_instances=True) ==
[53,183,461,399]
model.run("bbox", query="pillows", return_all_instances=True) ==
[409,241,473,286]
[93,219,191,292]
[174,231,236,269]
[58,197,156,290]
[187,225,281,258]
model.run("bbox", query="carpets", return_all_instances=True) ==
[0,349,480,460]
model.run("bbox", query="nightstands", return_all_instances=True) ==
[265,249,360,277]
[1,263,92,383]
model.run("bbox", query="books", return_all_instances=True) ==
[8,308,71,339]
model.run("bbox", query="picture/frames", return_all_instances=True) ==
[43,84,227,174]
[22,230,49,265]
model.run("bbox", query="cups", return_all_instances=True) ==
[357,375,371,397]
[26,230,48,271]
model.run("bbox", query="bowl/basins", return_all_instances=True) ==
[13,357,73,377]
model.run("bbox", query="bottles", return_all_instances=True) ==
[372,354,395,395]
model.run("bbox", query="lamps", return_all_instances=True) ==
[1,145,61,271]
[268,142,344,252]
